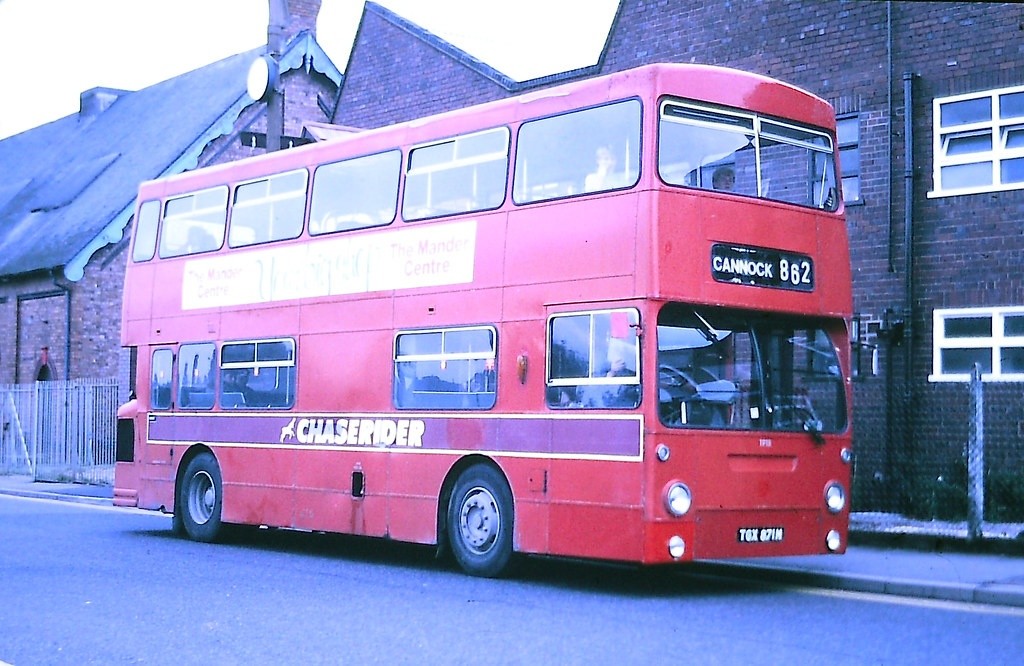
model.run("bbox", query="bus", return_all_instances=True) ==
[110,64,869,579]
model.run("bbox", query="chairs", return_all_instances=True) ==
[174,165,589,268]
[170,367,718,429]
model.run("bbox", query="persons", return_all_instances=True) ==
[713,166,736,192]
[584,145,621,190]
[599,336,635,379]
